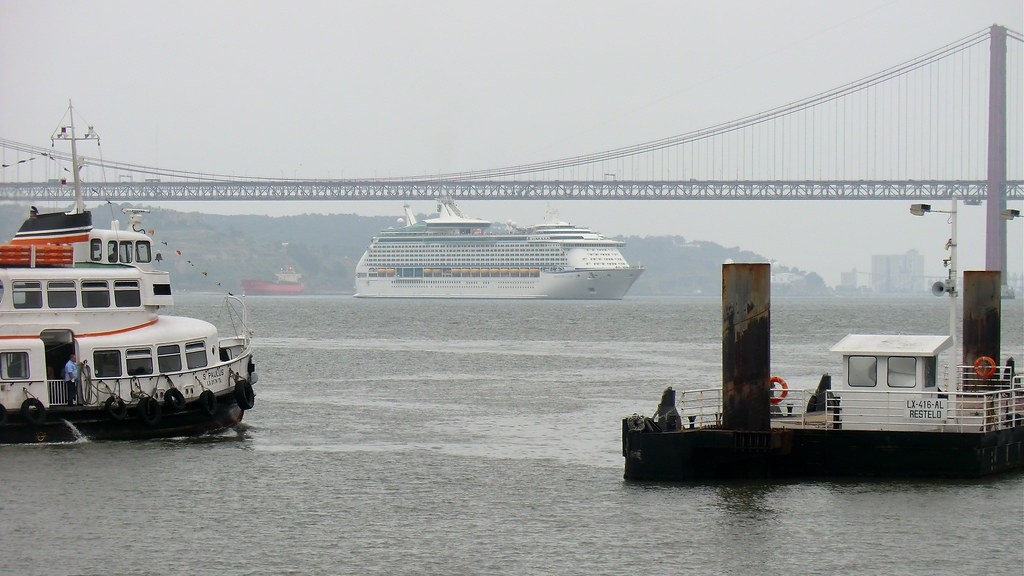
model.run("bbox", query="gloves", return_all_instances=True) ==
[71,378,75,383]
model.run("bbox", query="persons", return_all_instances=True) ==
[107,246,123,262]
[65,354,78,406]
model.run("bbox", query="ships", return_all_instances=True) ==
[352,195,646,299]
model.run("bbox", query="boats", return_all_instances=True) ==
[481,269,489,275]
[377,269,385,275]
[531,269,540,275]
[242,272,307,296]
[490,269,500,275]
[424,269,432,275]
[452,269,461,275]
[0,99,261,449]
[471,269,479,275]
[501,269,509,275]
[510,269,519,275]
[387,269,394,275]
[620,197,1024,486]
[520,269,529,275]
[433,269,442,274]
[462,269,469,275]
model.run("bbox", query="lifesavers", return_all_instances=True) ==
[974,357,996,379]
[234,379,255,410]
[769,377,789,405]
[0,403,8,426]
[138,397,160,423]
[164,388,186,412]
[199,390,218,416]
[20,397,46,427]
[105,394,128,420]
